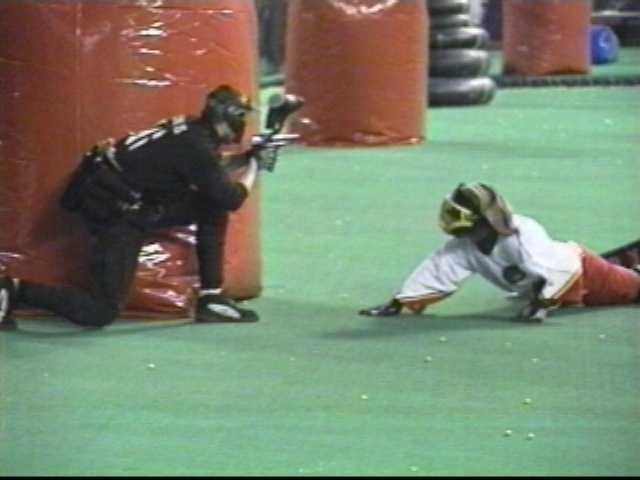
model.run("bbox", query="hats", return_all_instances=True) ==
[444,180,514,238]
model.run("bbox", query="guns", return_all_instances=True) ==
[248,94,305,170]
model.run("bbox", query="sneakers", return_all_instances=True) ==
[195,286,259,322]
[0,274,17,332]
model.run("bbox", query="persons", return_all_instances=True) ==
[357,182,639,324]
[1,85,277,328]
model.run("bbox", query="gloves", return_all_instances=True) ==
[519,302,548,322]
[360,299,403,317]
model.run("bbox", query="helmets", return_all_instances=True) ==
[209,84,256,110]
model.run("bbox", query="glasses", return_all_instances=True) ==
[435,193,481,233]
